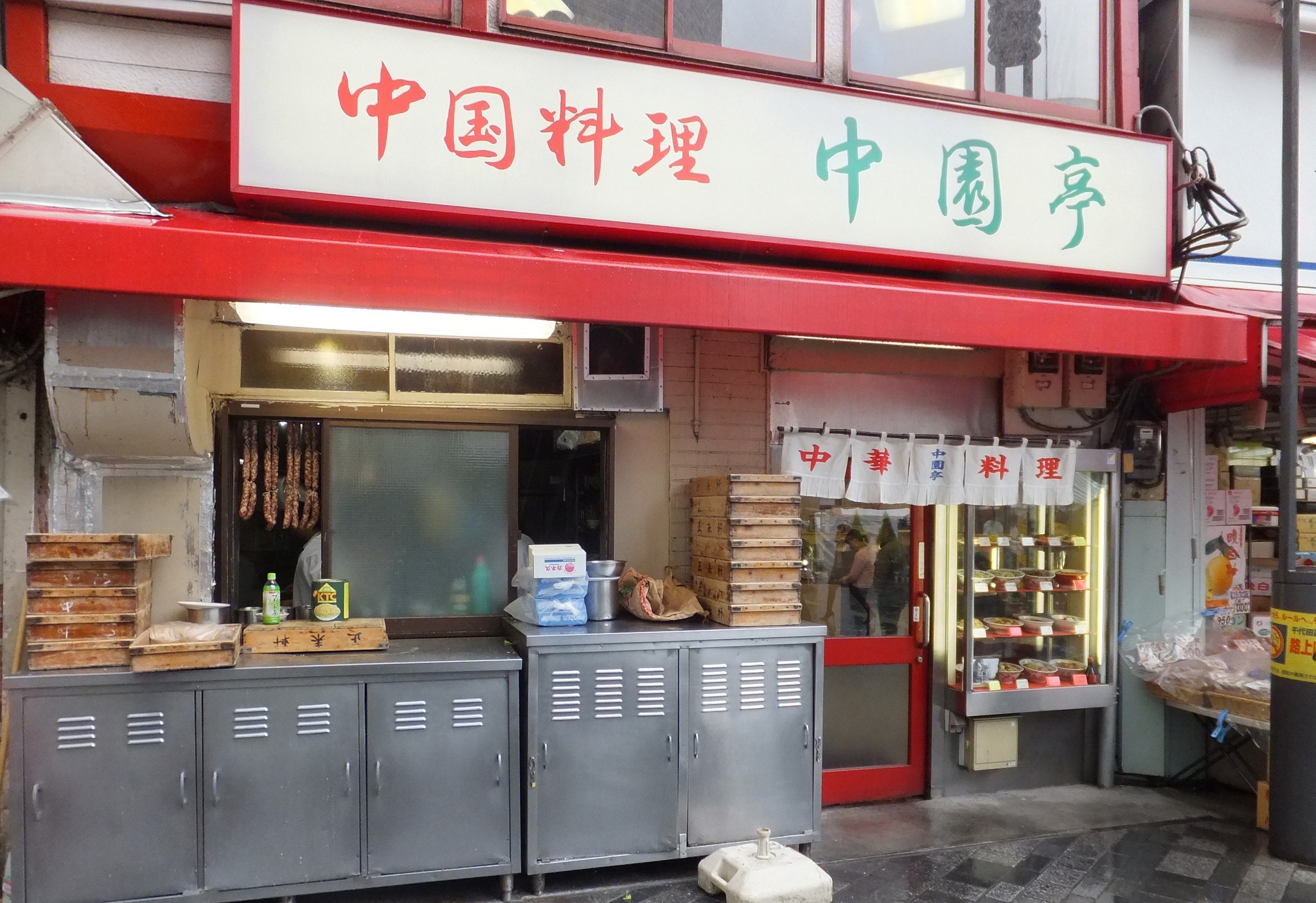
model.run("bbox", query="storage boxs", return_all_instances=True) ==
[527,544,588,626]
[1203,438,1316,639]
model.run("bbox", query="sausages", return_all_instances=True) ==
[238,420,320,532]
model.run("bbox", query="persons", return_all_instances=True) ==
[873,523,908,636]
[493,533,535,609]
[283,508,322,608]
[832,529,873,636]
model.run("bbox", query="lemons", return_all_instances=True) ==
[1206,557,1233,596]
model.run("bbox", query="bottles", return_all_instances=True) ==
[471,556,491,616]
[1085,658,1099,684]
[263,573,280,624]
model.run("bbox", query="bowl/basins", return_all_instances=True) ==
[1051,615,1086,632]
[1050,569,1088,588]
[313,604,341,621]
[983,617,1024,632]
[1018,615,1054,633]
[586,560,627,578]
[256,612,289,621]
[973,658,999,678]
[956,664,962,678]
[1017,568,1056,589]
[1049,659,1087,681]
[1019,659,1058,683]
[957,569,995,588]
[997,662,1024,684]
[987,568,1025,589]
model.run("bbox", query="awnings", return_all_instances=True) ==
[1264,326,1316,387]
[0,195,1263,413]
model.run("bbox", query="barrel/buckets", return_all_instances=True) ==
[584,581,619,620]
[294,605,311,619]
[235,606,263,628]
[187,607,230,624]
[280,607,294,620]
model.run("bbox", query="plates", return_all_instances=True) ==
[177,601,230,610]
[956,622,989,631]
[957,536,1073,546]
[589,576,620,581]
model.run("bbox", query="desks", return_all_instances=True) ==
[1164,699,1270,795]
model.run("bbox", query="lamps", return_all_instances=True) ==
[1246,399,1267,430]
[1298,387,1309,431]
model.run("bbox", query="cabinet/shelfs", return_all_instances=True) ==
[943,529,1111,719]
[3,613,827,903]
